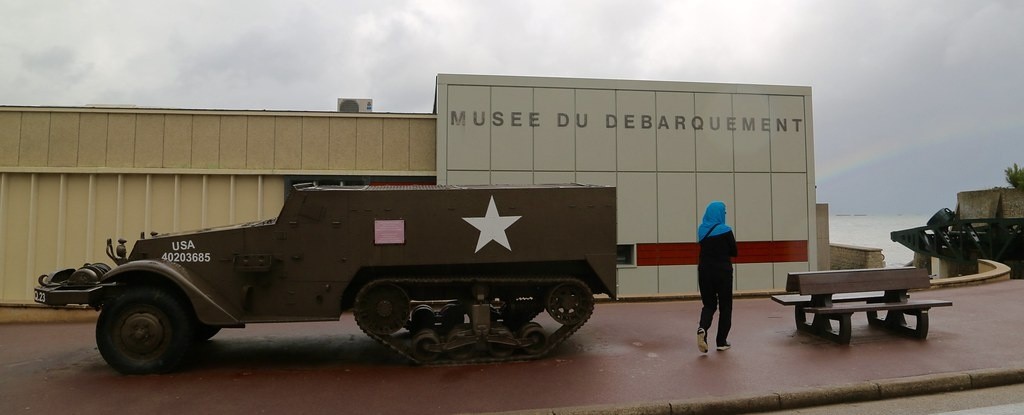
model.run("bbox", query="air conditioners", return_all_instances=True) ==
[337,97,373,112]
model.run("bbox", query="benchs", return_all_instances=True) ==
[798,268,952,347]
[771,267,916,334]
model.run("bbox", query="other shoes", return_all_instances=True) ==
[696,328,709,353]
[716,342,733,350]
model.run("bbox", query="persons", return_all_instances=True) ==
[696,200,739,354]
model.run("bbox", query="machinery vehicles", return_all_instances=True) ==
[32,181,620,375]
